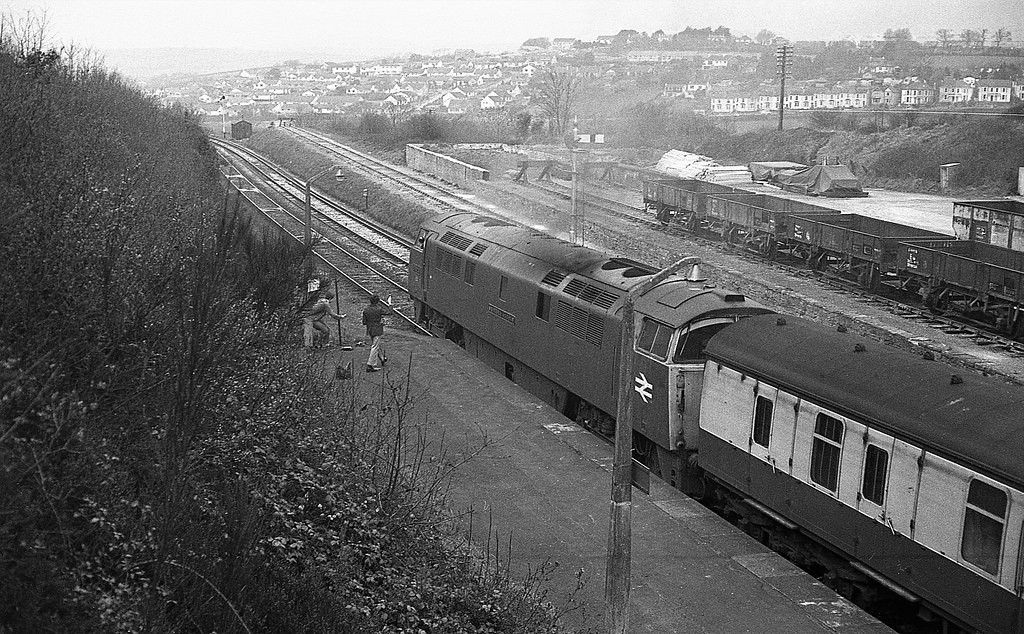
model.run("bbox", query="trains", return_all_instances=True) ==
[643,176,1024,340]
[407,207,1024,634]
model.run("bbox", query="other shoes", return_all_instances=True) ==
[367,364,381,372]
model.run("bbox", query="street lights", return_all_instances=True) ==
[600,253,707,633]
[303,163,345,304]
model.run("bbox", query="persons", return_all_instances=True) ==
[362,294,393,372]
[307,291,347,350]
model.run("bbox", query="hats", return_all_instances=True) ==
[370,295,379,304]
[325,293,335,300]
